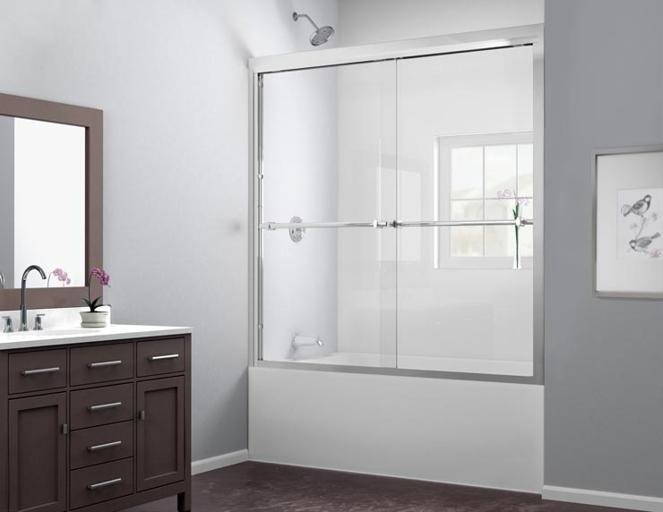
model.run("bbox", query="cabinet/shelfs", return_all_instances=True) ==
[0,335,187,511]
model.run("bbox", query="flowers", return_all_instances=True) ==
[45,268,73,290]
[77,265,110,312]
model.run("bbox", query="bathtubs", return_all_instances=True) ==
[286,353,533,377]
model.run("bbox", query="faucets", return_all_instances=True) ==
[19,265,47,331]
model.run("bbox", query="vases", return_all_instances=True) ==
[76,308,110,329]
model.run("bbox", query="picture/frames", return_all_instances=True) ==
[590,143,663,300]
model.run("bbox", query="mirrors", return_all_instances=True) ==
[0,92,103,309]
